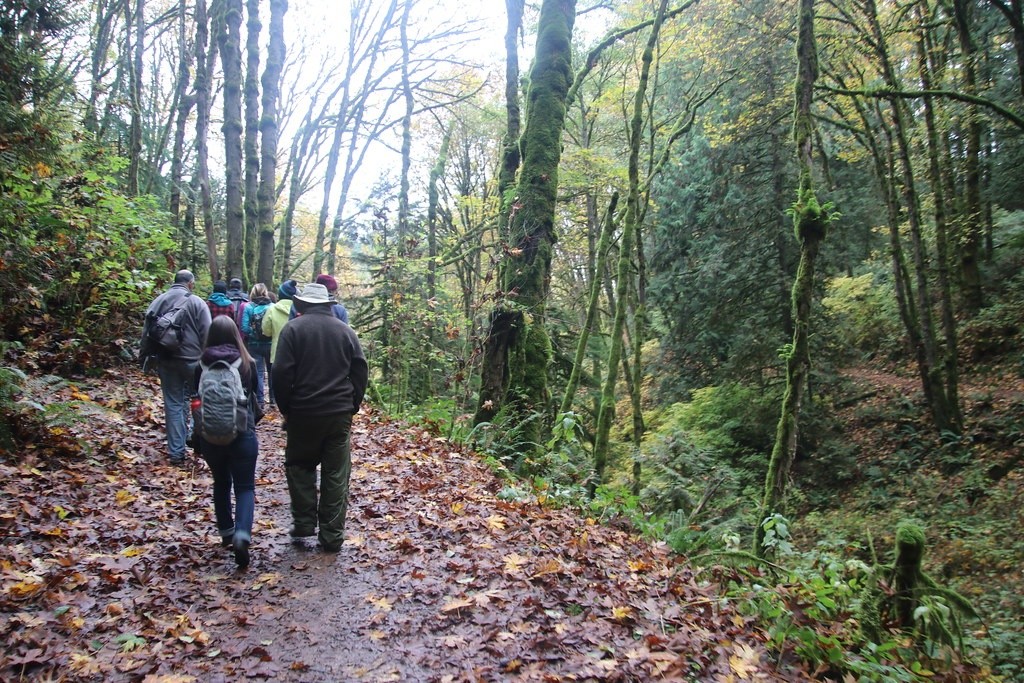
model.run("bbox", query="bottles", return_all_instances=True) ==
[143,354,154,376]
[190,393,201,410]
[238,394,247,412]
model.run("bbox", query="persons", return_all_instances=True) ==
[271,285,367,553]
[207,275,347,411]
[139,269,213,464]
[194,315,265,566]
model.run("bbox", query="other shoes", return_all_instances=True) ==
[258,403,264,410]
[288,524,317,538]
[221,534,233,550]
[323,545,341,552]
[170,461,186,471]
[234,538,249,566]
[187,436,201,454]
[270,400,276,407]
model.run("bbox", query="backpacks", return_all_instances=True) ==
[249,302,272,341]
[192,356,248,444]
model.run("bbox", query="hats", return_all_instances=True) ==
[214,280,226,294]
[278,279,297,298]
[315,274,338,290]
[293,283,338,313]
[231,278,241,289]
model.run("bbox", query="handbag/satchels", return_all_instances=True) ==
[147,292,193,353]
